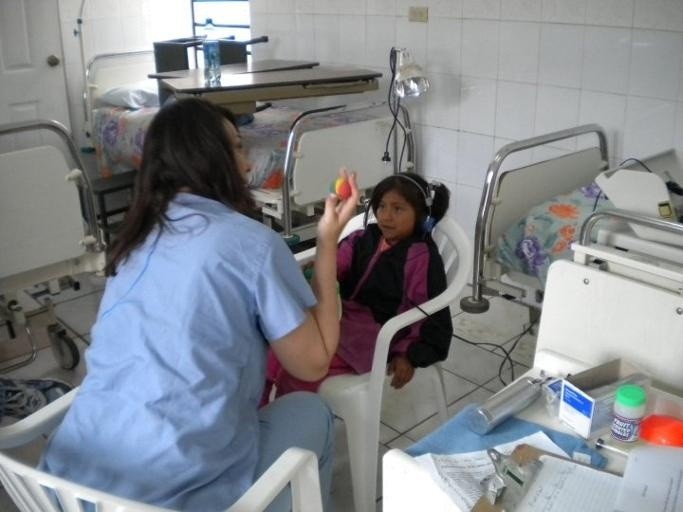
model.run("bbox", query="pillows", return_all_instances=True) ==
[98,84,159,111]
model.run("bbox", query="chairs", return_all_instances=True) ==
[266,207,472,512]
[0,364,326,512]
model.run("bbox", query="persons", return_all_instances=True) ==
[32,96,337,512]
[258,170,454,409]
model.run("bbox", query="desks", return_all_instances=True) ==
[149,59,383,123]
[383,209,682,512]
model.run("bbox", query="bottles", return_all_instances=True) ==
[610,384,647,442]
[464,375,546,437]
[202,18,221,83]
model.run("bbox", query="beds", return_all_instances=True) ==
[460,123,682,326]
[84,47,415,258]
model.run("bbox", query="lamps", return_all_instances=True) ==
[387,47,430,175]
